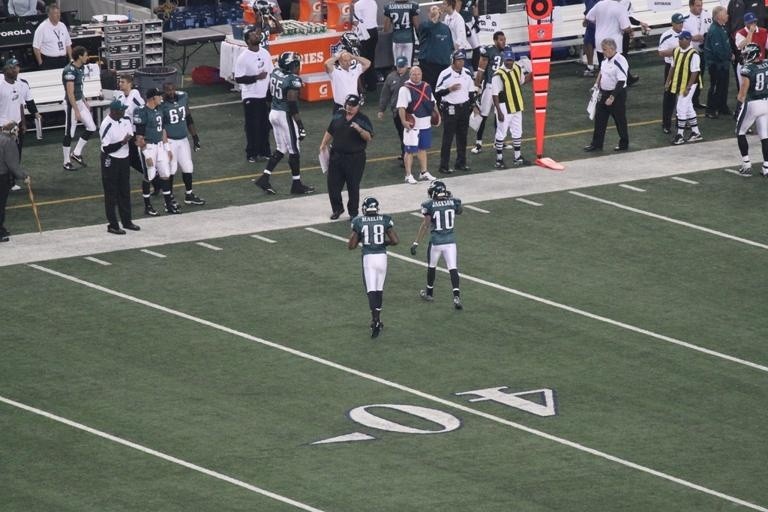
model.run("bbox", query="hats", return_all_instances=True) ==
[109,100,129,111]
[395,57,407,67]
[345,95,360,105]
[675,31,693,38]
[6,58,20,65]
[145,88,165,96]
[503,51,515,60]
[743,12,758,23]
[672,13,685,23]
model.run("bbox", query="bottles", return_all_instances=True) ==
[127,9,132,21]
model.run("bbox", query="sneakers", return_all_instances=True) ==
[420,290,434,300]
[10,185,22,191]
[584,144,600,150]
[453,296,463,308]
[739,164,752,175]
[331,210,344,219]
[695,102,736,119]
[292,185,316,194]
[665,121,704,144]
[123,223,140,230]
[615,145,625,149]
[144,175,205,216]
[256,179,276,195]
[471,144,529,168]
[247,153,272,163]
[760,167,768,176]
[370,320,384,337]
[69,152,87,167]
[108,227,126,235]
[63,162,76,171]
[405,165,471,184]
[629,75,639,87]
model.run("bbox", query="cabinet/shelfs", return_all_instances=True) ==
[79,17,164,78]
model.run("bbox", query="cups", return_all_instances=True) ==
[280,19,328,36]
[641,27,649,35]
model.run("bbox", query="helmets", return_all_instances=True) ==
[449,51,465,65]
[278,52,301,74]
[361,197,379,215]
[741,43,762,62]
[427,180,447,199]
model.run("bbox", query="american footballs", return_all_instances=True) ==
[431,110,439,125]
[406,111,415,128]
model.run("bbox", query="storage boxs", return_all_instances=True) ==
[299,72,334,103]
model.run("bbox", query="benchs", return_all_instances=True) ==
[0,62,116,141]
[481,0,731,54]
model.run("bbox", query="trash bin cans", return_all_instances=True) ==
[135,66,177,97]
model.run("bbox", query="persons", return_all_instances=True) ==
[378,2,532,184]
[319,94,374,220]
[347,195,401,338]
[32,5,72,71]
[2,118,31,241]
[324,50,372,149]
[99,74,205,234]
[254,51,316,194]
[334,32,368,107]
[62,45,97,171]
[8,2,46,17]
[242,2,282,52]
[350,1,378,91]
[410,179,465,311]
[234,30,274,163]
[2,58,41,191]
[577,0,768,180]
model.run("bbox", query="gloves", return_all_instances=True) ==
[192,135,201,152]
[297,121,307,140]
[411,244,419,254]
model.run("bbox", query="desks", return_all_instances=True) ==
[160,26,226,76]
[219,25,349,92]
